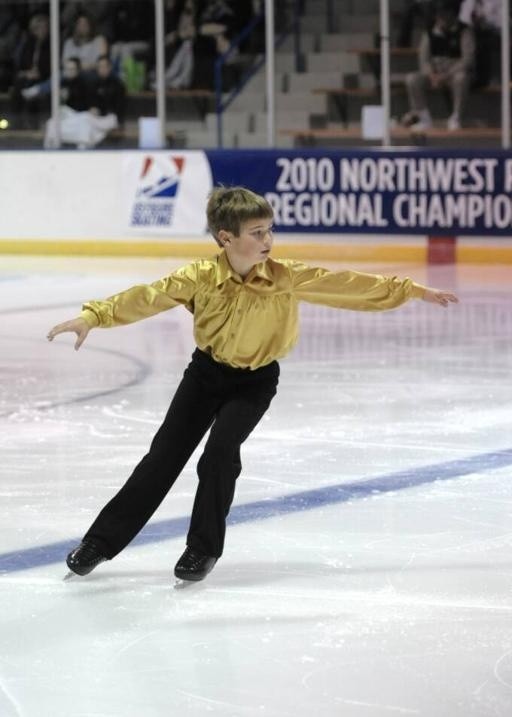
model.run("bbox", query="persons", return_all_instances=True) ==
[401,1,503,134]
[0,0,265,149]
[45,185,462,582]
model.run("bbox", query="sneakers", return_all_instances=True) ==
[66,542,109,575]
[174,545,218,581]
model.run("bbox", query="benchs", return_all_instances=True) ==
[0,1,512,147]
